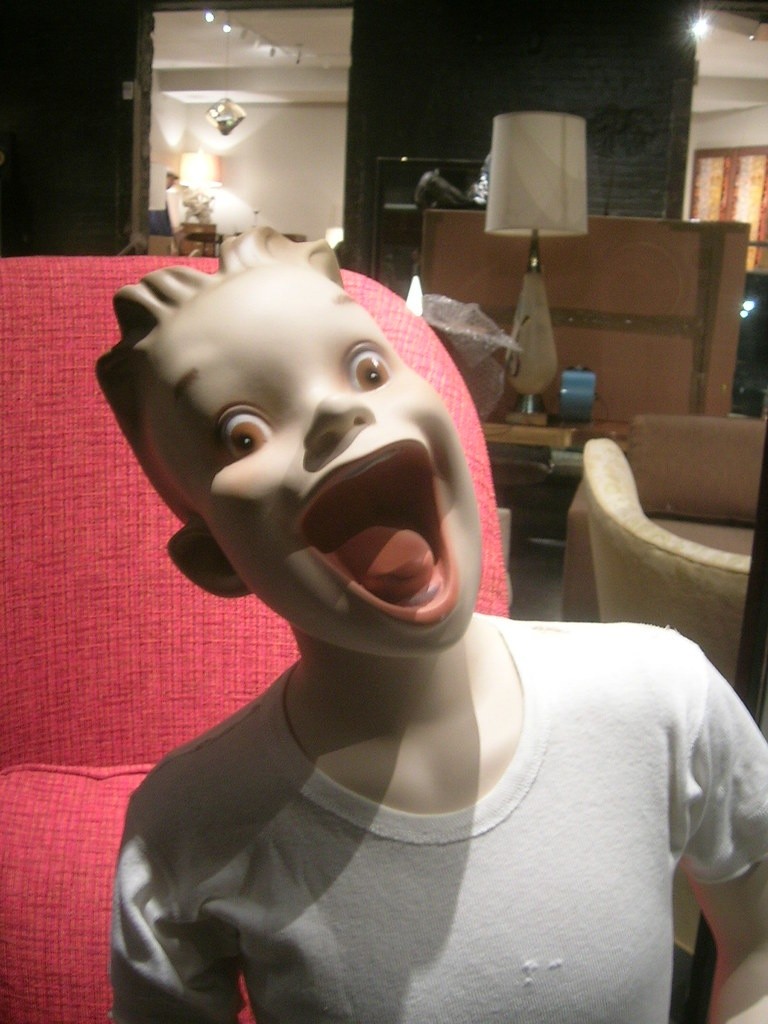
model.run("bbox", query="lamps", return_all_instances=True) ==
[484,112,588,427]
[206,12,246,134]
[178,153,224,224]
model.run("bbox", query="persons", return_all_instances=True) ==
[93,228,768,1024]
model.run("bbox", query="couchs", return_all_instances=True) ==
[582,439,768,690]
[560,415,768,634]
[0,259,508,1024]
